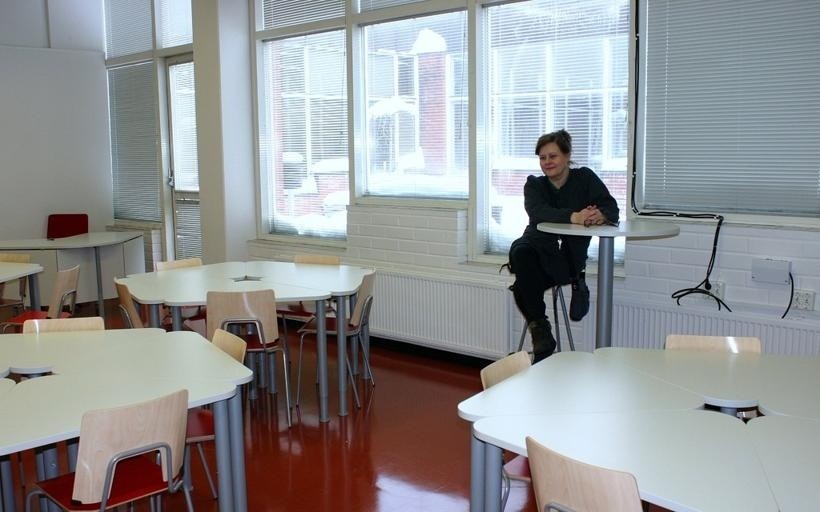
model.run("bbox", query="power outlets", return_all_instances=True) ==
[703,282,725,301]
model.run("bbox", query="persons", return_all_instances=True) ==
[509,130,619,365]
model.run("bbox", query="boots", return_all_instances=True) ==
[527,318,555,366]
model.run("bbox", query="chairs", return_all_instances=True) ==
[526,436,643,512]
[481,349,532,512]
[664,334,761,354]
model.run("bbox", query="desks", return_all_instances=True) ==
[458,347,820,512]
[537,220,680,349]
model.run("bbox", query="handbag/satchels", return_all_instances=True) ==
[570,279,590,320]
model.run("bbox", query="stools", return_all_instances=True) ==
[517,278,576,351]
